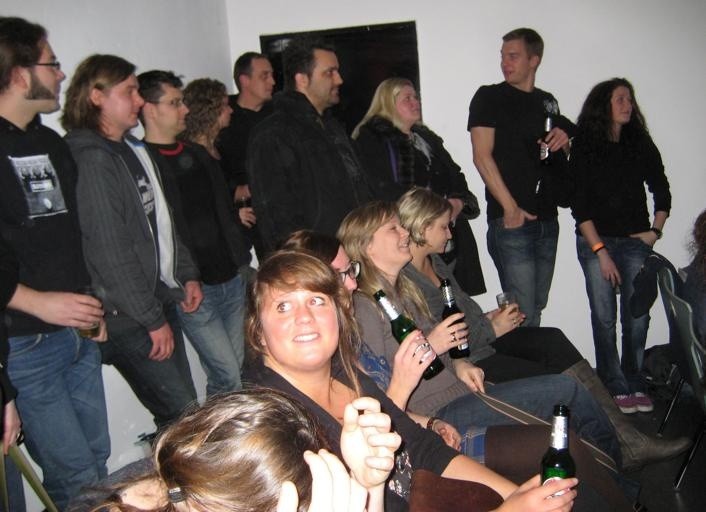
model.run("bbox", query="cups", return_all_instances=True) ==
[234,197,252,209]
[495,292,519,315]
[72,285,103,338]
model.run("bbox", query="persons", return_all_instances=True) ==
[62,55,204,427]
[87,29,705,511]
[0,325,21,512]
[0,18,107,512]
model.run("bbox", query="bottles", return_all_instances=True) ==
[540,403,577,500]
[540,111,555,166]
[373,289,445,380]
[441,278,470,359]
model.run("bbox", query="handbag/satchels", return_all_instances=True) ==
[641,345,704,439]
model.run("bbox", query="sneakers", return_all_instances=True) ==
[615,387,655,414]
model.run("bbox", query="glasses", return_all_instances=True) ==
[336,259,363,283]
[33,60,62,68]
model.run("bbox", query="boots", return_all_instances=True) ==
[562,359,695,468]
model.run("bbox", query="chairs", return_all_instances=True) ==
[647,250,706,491]
